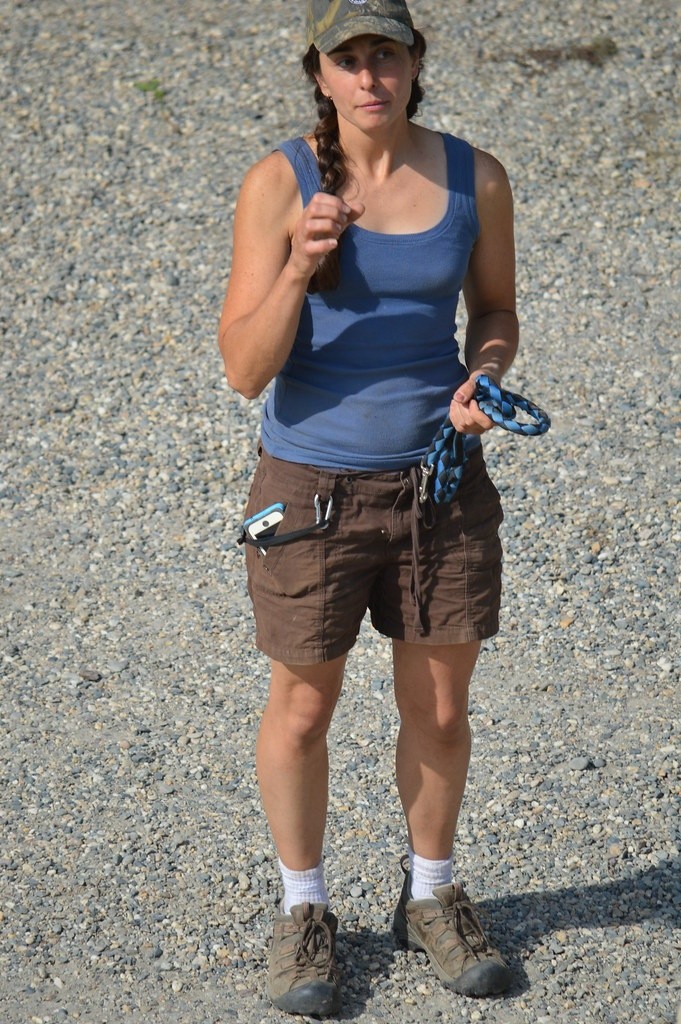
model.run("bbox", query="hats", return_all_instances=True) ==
[302,0,414,59]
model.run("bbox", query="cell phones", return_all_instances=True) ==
[243,502,285,557]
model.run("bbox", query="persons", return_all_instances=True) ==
[217,0,522,1015]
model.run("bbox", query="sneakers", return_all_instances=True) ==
[267,898,345,1015]
[392,855,512,996]
